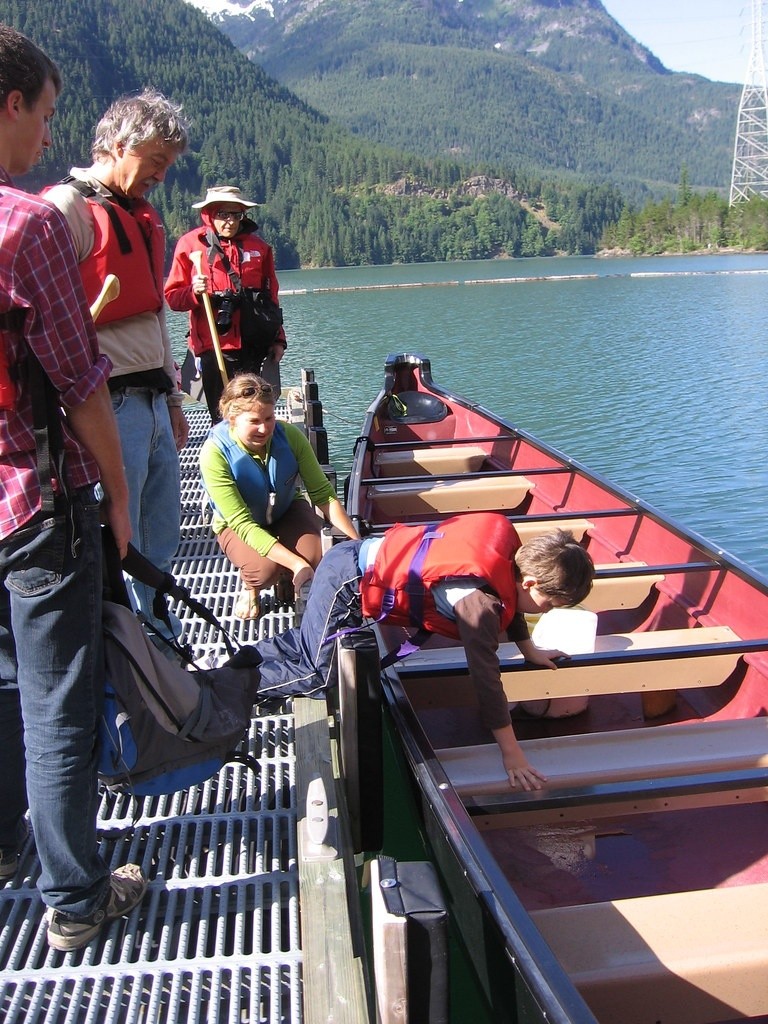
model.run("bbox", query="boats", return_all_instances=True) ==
[337,349,767,1023]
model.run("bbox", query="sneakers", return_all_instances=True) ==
[48,862,147,951]
[0,815,28,875]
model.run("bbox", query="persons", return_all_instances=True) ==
[198,374,359,620]
[41,91,189,644]
[164,185,287,423]
[0,26,149,949]
[188,513,595,791]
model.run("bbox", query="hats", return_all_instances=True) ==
[192,186,257,209]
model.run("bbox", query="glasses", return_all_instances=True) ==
[215,211,244,220]
[236,384,272,398]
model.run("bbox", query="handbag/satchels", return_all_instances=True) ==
[241,287,284,349]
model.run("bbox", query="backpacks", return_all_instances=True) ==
[101,533,261,805]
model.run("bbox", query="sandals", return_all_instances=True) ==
[234,584,259,622]
